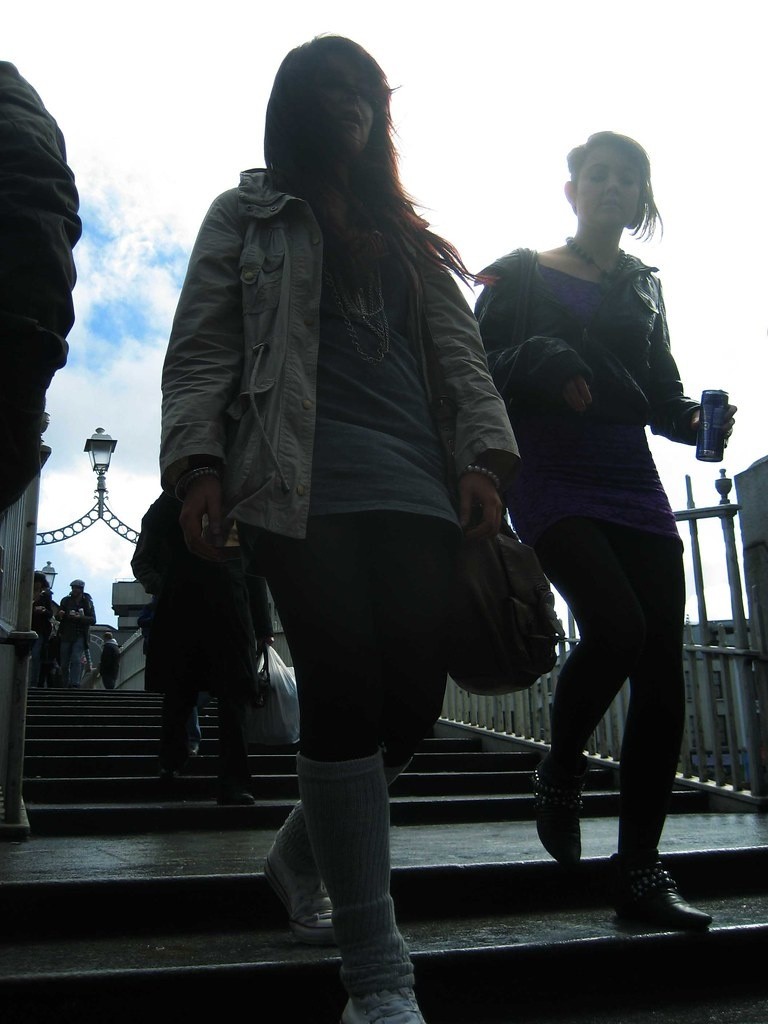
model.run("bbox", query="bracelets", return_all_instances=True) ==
[174,467,223,502]
[467,464,501,490]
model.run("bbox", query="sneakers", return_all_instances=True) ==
[340,988,426,1024]
[265,840,338,945]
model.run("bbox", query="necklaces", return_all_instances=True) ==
[564,236,628,283]
[324,246,393,368]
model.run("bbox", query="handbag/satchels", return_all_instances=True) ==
[447,516,569,695]
[241,643,300,745]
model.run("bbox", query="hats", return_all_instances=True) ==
[70,580,84,587]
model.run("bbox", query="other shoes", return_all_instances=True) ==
[217,789,255,804]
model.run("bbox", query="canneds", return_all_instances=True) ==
[696,390,728,462]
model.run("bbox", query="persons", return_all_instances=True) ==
[162,34,525,1016]
[0,61,82,511]
[470,133,735,932]
[130,489,275,805]
[32,572,121,687]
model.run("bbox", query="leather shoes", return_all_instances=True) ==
[532,753,588,867]
[608,853,712,927]
